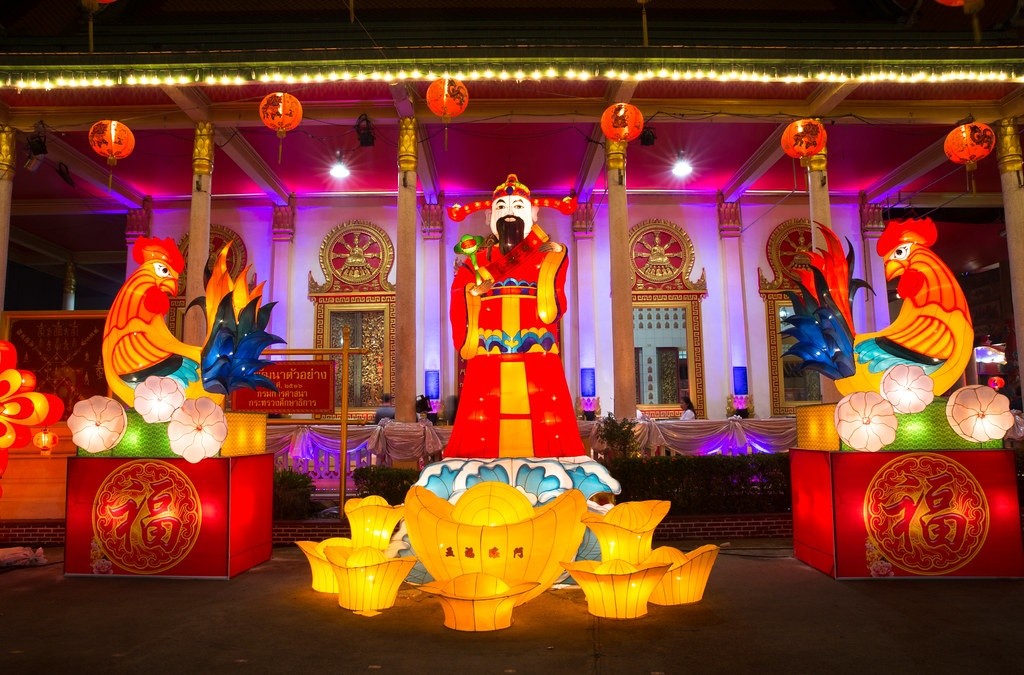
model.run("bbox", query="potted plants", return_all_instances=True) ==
[273,465,317,519]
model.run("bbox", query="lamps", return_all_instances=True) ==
[27,136,49,155]
[358,129,377,146]
[638,127,657,146]
[780,306,787,320]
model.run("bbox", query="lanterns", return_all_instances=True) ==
[780,118,828,168]
[425,77,469,124]
[88,120,135,168]
[258,85,303,138]
[943,122,996,170]
[600,102,645,169]
[987,376,1005,392]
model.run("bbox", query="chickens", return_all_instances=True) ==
[101,234,288,408]
[776,216,974,397]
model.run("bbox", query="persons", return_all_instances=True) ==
[374,393,395,466]
[1009,385,1023,413]
[442,174,586,458]
[679,396,697,420]
